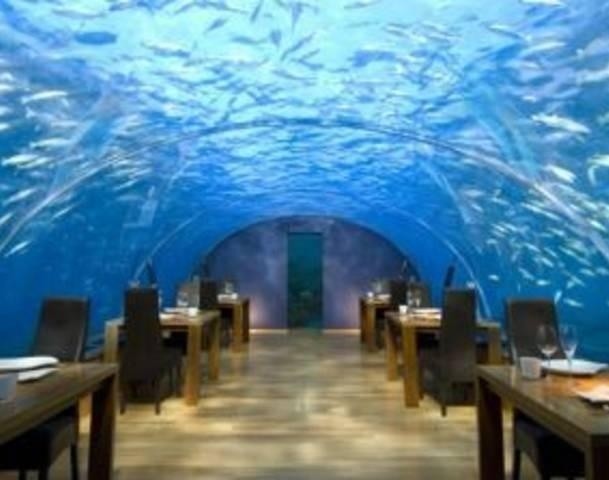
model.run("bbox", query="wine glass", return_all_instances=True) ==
[536,324,578,376]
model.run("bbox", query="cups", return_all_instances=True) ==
[398,304,408,315]
[519,356,544,380]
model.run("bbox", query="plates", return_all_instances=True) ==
[0,356,58,371]
[413,308,441,314]
[542,357,606,376]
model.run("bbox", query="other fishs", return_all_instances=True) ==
[0,0,609,308]
